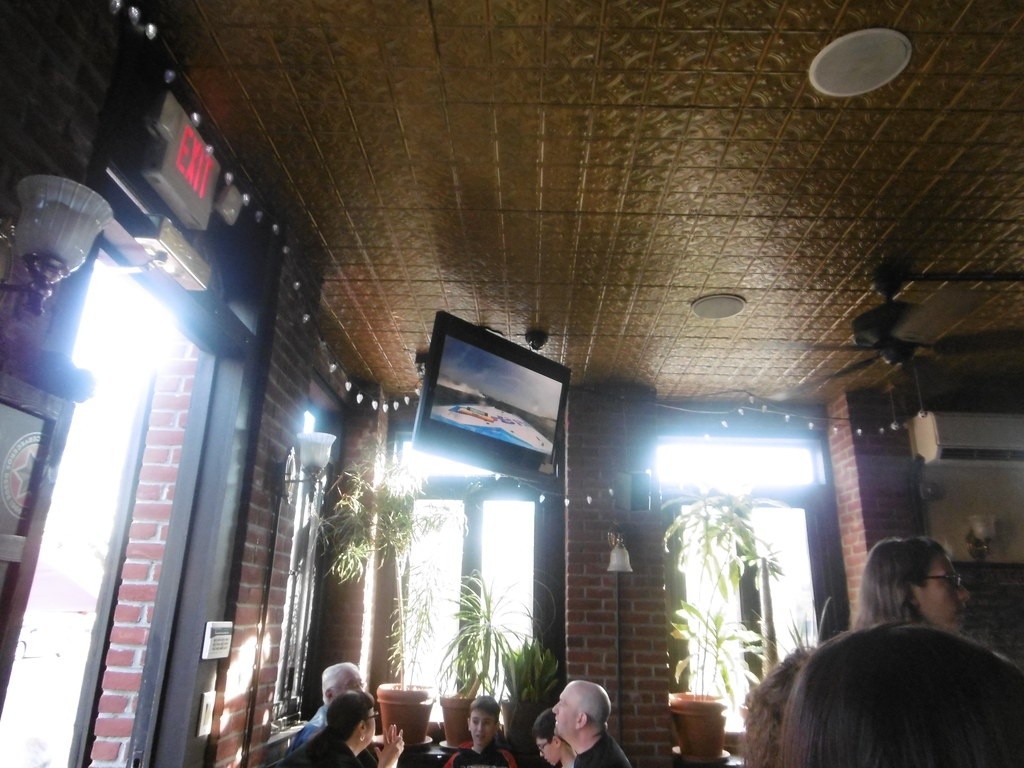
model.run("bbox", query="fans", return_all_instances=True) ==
[790,269,1024,394]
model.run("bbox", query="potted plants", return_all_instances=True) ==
[663,487,785,768]
[437,569,519,746]
[499,640,558,752]
[319,444,443,743]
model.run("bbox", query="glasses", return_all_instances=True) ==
[536,738,555,753]
[363,711,380,720]
[920,573,962,587]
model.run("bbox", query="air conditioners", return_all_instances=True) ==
[910,410,1024,469]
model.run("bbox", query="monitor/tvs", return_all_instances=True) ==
[412,310,573,483]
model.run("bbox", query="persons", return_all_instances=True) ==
[275,662,404,768]
[746,621,1024,768]
[532,708,577,768]
[552,680,631,768]
[857,538,970,631]
[443,696,523,768]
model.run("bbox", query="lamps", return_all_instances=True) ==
[277,431,337,503]
[606,522,634,573]
[0,172,113,316]
